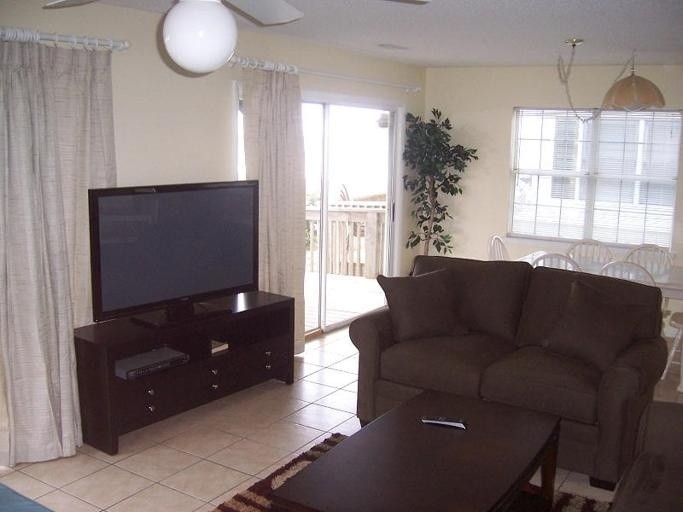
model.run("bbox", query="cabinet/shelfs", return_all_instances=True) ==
[74,291,294,456]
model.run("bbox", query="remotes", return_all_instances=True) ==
[421,415,467,430]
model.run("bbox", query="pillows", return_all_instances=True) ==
[543,278,656,373]
[376,266,469,341]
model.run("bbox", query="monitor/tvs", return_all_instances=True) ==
[88,180,259,328]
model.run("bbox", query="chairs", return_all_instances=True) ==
[488,234,672,309]
[660,312,683,394]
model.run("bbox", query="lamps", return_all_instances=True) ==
[161,0,236,74]
[601,52,666,112]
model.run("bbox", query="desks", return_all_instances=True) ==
[514,250,683,302]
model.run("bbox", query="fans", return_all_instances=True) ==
[42,0,304,26]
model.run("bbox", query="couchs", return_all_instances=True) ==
[611,400,682,511]
[349,255,667,491]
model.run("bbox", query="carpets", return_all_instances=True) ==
[209,432,612,512]
[0,483,52,511]
